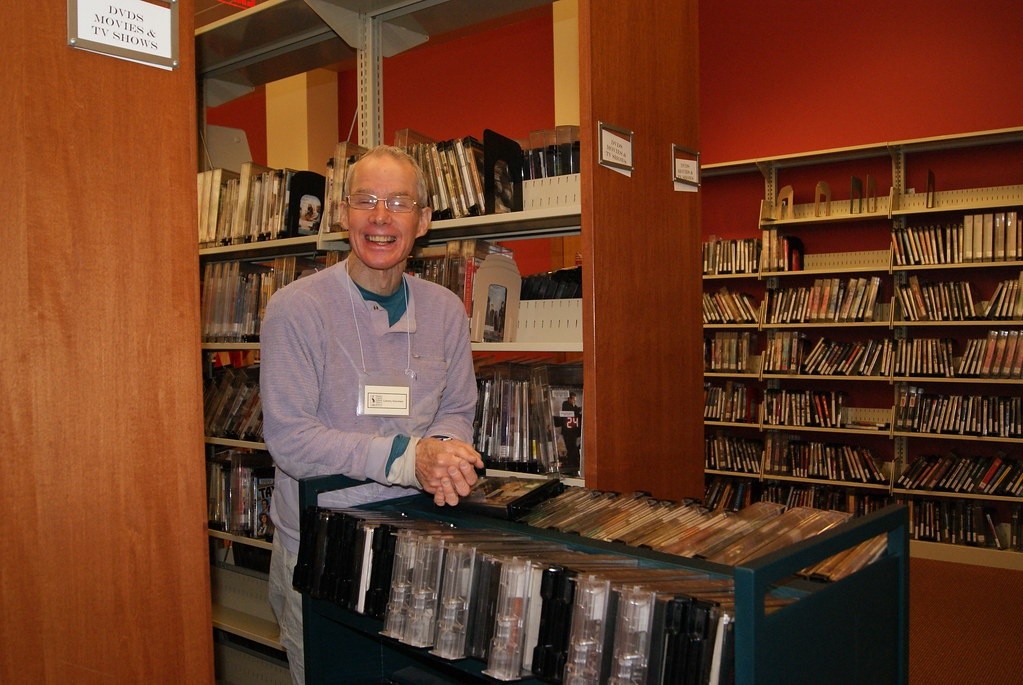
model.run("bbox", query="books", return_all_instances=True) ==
[473,361,583,479]
[895,270,1023,495]
[197,157,334,250]
[207,450,276,541]
[703,229,805,276]
[203,377,266,441]
[200,258,325,343]
[702,276,894,485]
[392,125,579,221]
[892,212,1023,267]
[407,237,513,342]
[705,475,1023,553]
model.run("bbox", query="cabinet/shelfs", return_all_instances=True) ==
[1,0,1023,685]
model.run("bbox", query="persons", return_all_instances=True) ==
[499,301,505,337]
[258,499,269,518]
[494,312,498,337]
[258,514,269,537]
[260,146,484,685]
[562,393,582,470]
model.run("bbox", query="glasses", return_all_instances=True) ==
[345,193,425,213]
